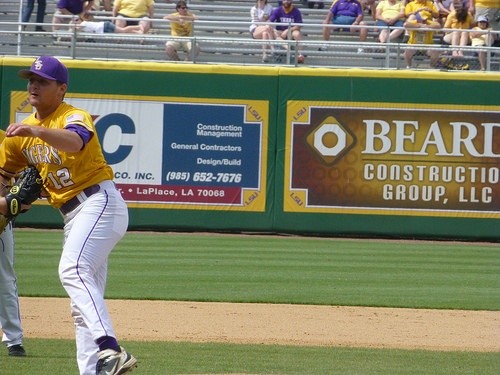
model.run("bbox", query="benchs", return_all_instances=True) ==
[0,0,500,70]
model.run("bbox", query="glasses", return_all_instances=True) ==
[180,6,189,9]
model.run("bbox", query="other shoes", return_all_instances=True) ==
[298,55,304,63]
[357,48,364,53]
[320,45,329,50]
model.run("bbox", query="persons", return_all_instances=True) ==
[318,0,368,54]
[358,0,500,71]
[1,55,139,375]
[51,0,156,34]
[17,0,47,34]
[0,129,27,356]
[163,0,200,61]
[249,0,307,65]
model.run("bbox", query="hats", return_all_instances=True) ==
[18,55,68,83]
[477,15,487,22]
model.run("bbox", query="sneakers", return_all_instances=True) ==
[8,345,26,356]
[262,54,268,63]
[96,345,138,375]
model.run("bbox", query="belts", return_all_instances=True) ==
[59,183,100,215]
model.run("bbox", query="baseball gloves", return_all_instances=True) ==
[4,164,43,220]
